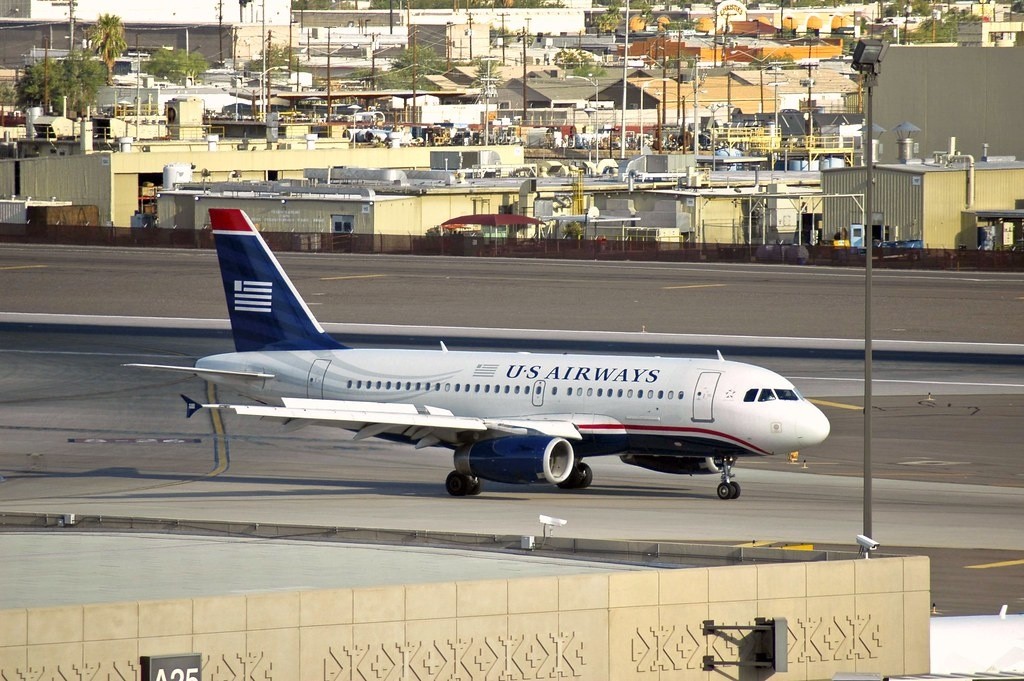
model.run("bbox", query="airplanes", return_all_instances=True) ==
[113,208,832,507]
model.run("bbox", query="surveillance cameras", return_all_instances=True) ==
[857,535,880,551]
[540,515,566,527]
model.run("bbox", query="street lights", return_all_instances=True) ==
[731,43,794,117]
[639,75,673,154]
[350,105,362,150]
[300,43,360,137]
[567,75,599,165]
[261,63,288,123]
